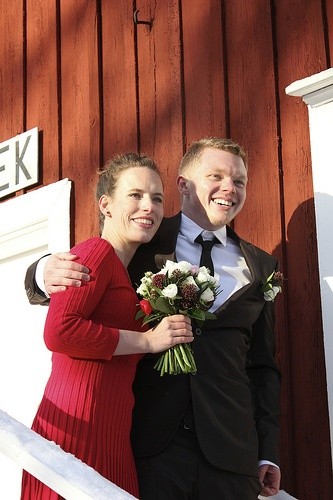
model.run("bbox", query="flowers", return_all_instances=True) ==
[260,270,288,304]
[134,260,223,377]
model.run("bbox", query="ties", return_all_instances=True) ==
[193,234,221,278]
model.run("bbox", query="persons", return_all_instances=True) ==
[23,137,285,500]
[20,152,196,500]
